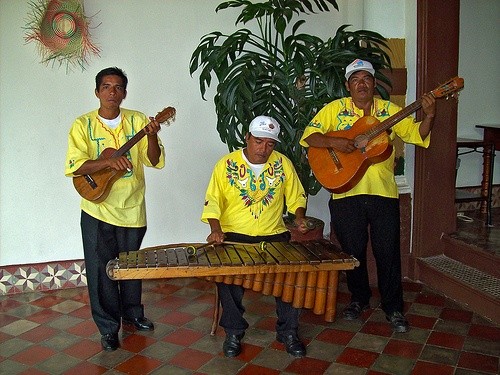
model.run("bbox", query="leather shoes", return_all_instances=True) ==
[121,316,154,331]
[223,330,245,357]
[275,328,307,356]
[385,311,409,333]
[101,331,120,352]
[342,299,371,320]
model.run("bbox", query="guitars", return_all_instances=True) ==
[72,106,176,205]
[309,77,465,195]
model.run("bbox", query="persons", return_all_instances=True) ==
[201,116,309,358]
[65,67,166,351]
[299,59,436,332]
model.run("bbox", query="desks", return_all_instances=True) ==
[455,138,496,228]
[475,124,500,241]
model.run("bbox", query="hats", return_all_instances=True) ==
[345,59,375,81]
[249,116,282,144]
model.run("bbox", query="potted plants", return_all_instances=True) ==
[189,0,395,241]
